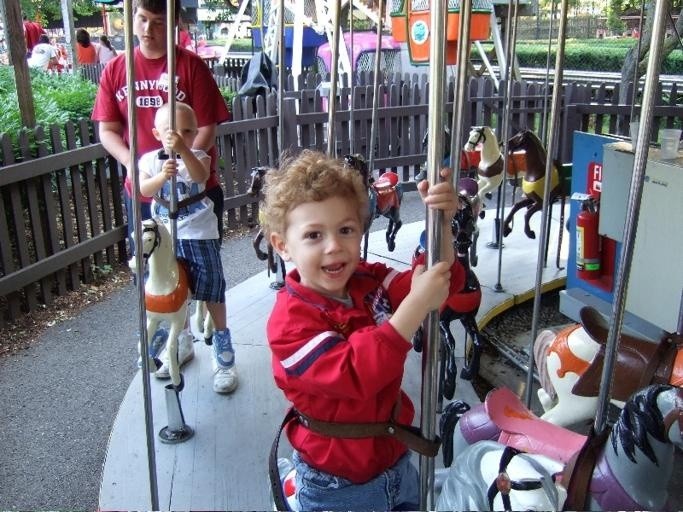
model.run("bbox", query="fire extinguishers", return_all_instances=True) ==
[576,197,603,280]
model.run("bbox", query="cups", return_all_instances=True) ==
[658,127,681,163]
[629,120,641,155]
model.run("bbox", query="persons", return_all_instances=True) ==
[96,35,117,65]
[258,144,465,510]
[176,14,195,53]
[74,27,95,64]
[90,0,240,396]
[28,33,58,75]
[137,103,237,369]
[21,15,46,59]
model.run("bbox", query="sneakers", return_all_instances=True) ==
[154,329,195,381]
[212,328,237,395]
